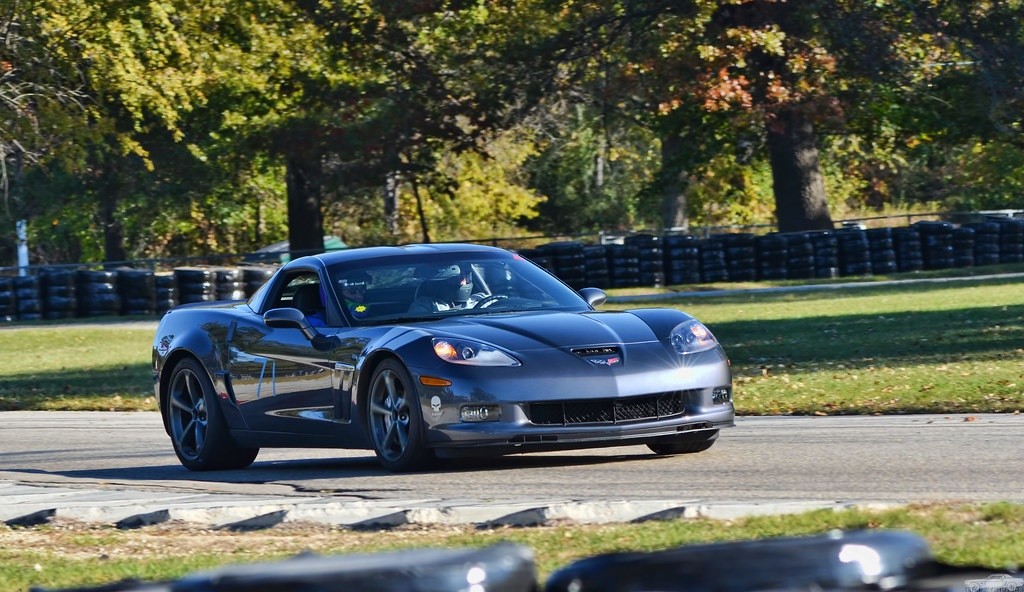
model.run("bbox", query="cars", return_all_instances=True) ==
[236,235,349,267]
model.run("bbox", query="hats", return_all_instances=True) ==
[343,272,368,287]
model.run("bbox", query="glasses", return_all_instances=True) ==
[342,287,367,296]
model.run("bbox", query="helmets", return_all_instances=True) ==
[433,262,473,303]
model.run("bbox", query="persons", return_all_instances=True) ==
[306,269,383,327]
[406,259,497,315]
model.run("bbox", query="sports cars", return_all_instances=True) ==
[152,250,732,474]
